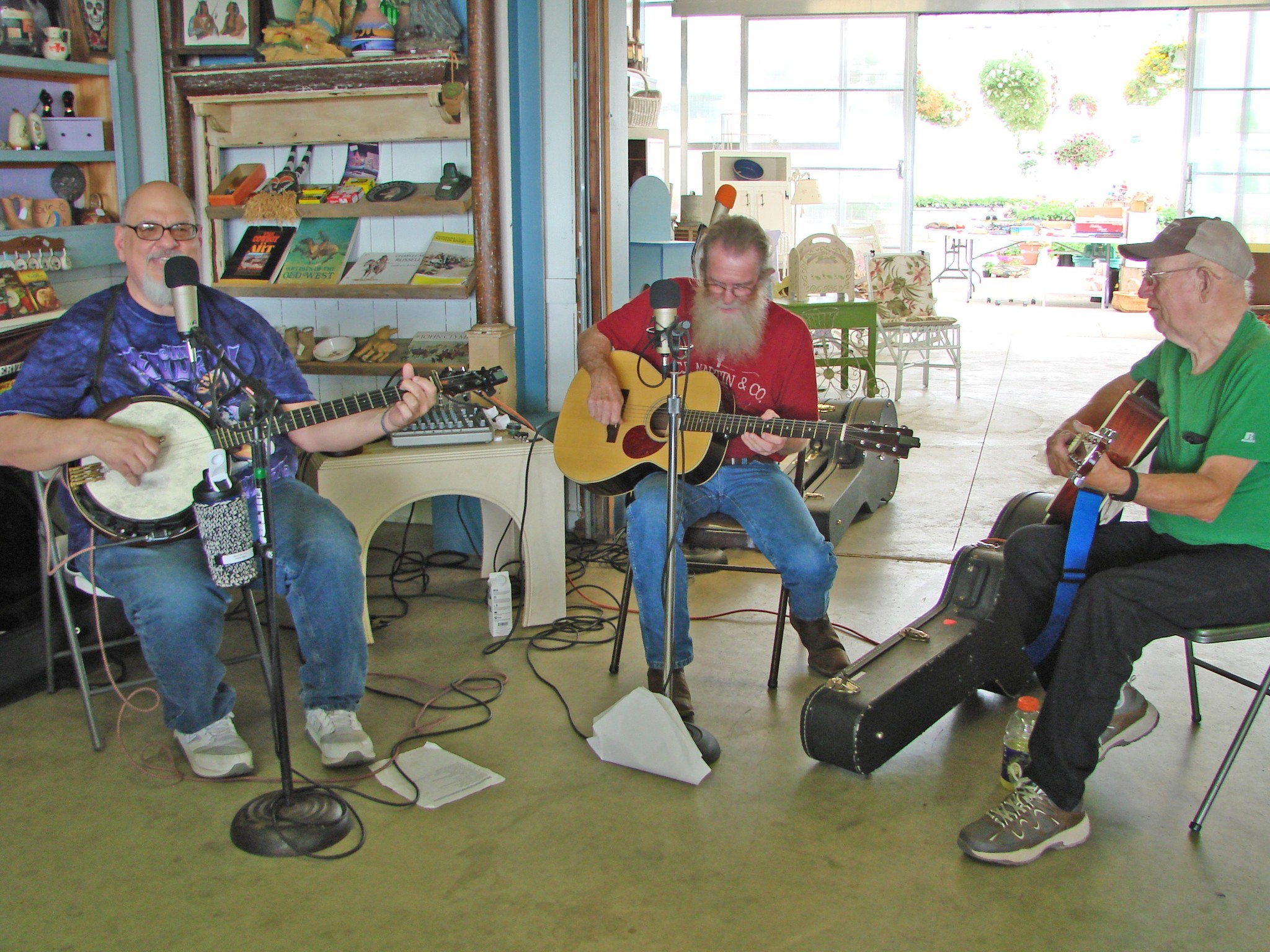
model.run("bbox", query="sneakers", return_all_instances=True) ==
[958,762,1090,866]
[174,711,254,779]
[305,707,375,767]
[1097,674,1159,762]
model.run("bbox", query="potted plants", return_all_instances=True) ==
[982,262,993,277]
[1041,208,1074,229]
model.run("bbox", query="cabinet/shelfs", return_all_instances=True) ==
[703,150,792,232]
[158,0,503,377]
[0,0,141,270]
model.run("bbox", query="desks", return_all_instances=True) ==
[952,233,1126,309]
[771,295,877,398]
[302,422,567,645]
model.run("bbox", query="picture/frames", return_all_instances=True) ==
[170,0,261,56]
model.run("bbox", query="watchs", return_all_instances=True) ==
[1108,466,1138,502]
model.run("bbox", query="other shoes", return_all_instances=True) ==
[789,603,851,677]
[647,667,695,717]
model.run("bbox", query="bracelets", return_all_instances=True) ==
[381,407,397,433]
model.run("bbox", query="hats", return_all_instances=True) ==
[1118,217,1254,279]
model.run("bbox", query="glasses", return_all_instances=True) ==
[1141,266,1220,286]
[125,222,198,241]
[704,269,759,297]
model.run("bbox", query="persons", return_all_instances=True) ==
[575,216,851,718]
[957,215,1270,867]
[1,180,437,781]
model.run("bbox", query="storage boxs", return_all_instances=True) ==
[344,177,375,193]
[1071,207,1124,233]
[326,186,365,203]
[208,162,266,206]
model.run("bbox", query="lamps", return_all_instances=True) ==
[791,173,826,248]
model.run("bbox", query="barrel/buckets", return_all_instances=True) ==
[1019,243,1122,269]
[681,191,702,223]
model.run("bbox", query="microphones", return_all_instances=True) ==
[649,280,681,379]
[164,256,200,374]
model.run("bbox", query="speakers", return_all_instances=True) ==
[0,460,139,706]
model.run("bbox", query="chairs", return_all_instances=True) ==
[863,252,961,400]
[609,448,807,689]
[1176,623,1270,831]
[831,224,883,299]
[32,465,272,749]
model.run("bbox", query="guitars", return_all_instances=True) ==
[554,351,921,492]
[1042,373,1166,529]
[63,363,509,546]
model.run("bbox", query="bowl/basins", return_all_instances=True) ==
[313,334,356,364]
[733,159,764,181]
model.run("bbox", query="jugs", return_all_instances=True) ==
[41,26,71,61]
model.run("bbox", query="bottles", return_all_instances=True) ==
[1000,696,1040,793]
[192,448,258,586]
[350,0,396,58]
[7,89,75,151]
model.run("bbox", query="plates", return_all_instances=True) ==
[366,180,415,201]
